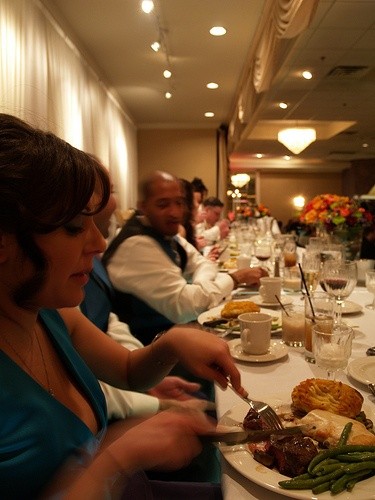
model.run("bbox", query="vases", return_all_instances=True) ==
[316,225,362,267]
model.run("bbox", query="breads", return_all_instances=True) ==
[292,378,364,418]
[222,258,239,268]
[222,301,261,319]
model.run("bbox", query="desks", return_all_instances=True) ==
[203,244,375,500]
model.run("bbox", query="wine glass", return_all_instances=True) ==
[321,259,358,331]
[254,240,273,269]
[363,269,375,310]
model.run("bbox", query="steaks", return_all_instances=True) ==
[243,407,269,429]
[253,428,317,476]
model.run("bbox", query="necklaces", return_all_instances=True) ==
[1,326,53,395]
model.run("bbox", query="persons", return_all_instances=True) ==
[180,177,231,262]
[100,171,271,347]
[80,166,219,427]
[0,113,248,500]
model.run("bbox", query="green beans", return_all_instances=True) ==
[212,322,282,331]
[278,422,375,495]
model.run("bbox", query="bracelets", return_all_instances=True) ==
[229,273,238,284]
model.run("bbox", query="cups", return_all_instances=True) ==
[225,207,361,373]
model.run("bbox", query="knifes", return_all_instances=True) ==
[199,423,310,445]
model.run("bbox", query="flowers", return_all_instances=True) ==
[300,192,372,227]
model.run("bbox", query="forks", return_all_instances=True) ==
[222,381,284,431]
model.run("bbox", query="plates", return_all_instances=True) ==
[217,397,375,500]
[349,354,375,389]
[309,296,363,314]
[197,303,283,337]
[223,336,288,362]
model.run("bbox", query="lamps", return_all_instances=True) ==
[278,120,316,155]
